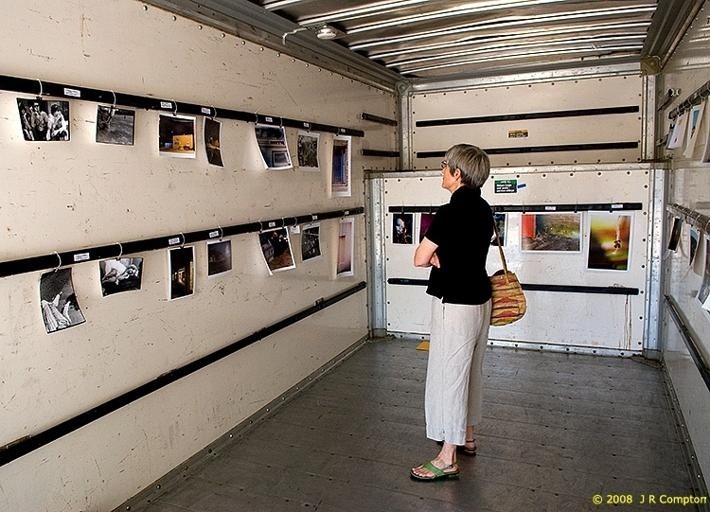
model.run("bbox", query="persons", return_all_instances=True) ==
[100,260,139,283]
[25,102,47,141]
[40,289,73,334]
[390,215,413,243]
[409,143,496,482]
[40,100,70,140]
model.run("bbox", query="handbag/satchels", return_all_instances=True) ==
[489,261,526,325]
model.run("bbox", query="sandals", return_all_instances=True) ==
[411,460,461,481]
[461,439,478,455]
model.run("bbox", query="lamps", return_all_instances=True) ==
[640,54,662,79]
[315,22,337,42]
